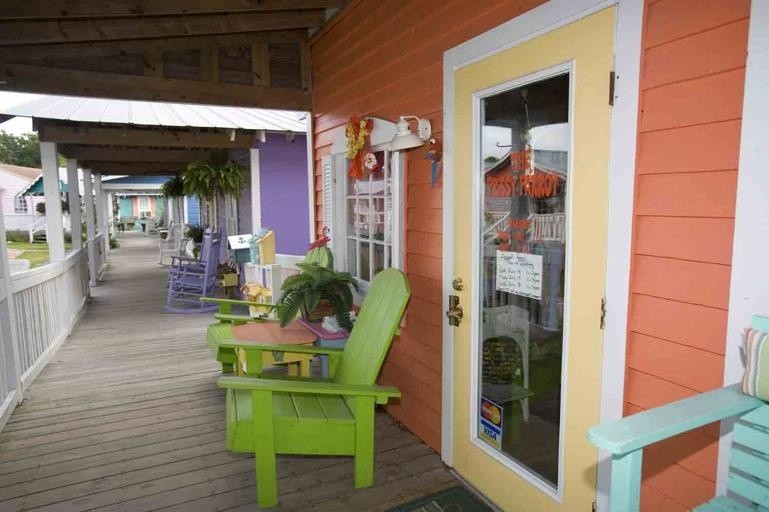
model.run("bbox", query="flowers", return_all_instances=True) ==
[208,248,242,280]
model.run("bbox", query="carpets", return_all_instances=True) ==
[385,486,494,512]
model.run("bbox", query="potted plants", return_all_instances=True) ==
[276,264,360,332]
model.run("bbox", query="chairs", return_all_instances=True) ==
[199,297,280,372]
[216,264,412,509]
[158,221,228,313]
[585,312,769,512]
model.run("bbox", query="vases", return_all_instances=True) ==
[222,273,239,287]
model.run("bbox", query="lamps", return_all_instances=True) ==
[387,116,432,151]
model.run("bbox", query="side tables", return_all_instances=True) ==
[231,320,317,378]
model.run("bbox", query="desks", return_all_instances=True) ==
[298,317,351,377]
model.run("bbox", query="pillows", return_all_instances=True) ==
[740,327,768,404]
